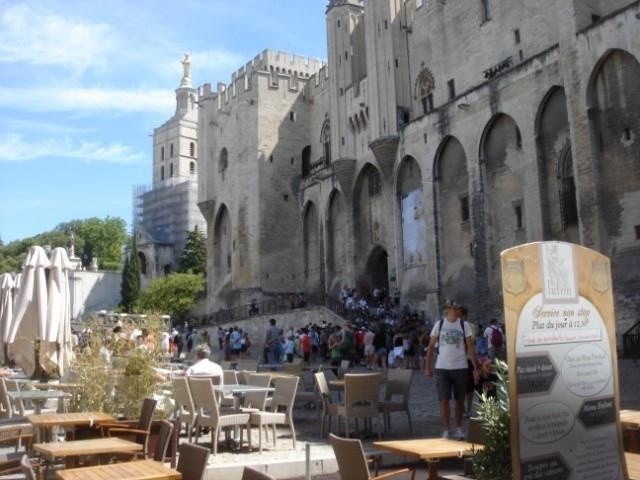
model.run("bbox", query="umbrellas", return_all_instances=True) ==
[1,244,75,408]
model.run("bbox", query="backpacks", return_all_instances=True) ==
[490,326,503,348]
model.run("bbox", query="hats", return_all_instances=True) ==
[442,299,462,310]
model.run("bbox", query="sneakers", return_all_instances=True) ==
[454,431,464,438]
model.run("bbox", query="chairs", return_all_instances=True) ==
[174,442,212,479]
[326,431,372,479]
[18,452,35,480]
[241,465,272,480]
[99,397,158,466]
[154,418,175,460]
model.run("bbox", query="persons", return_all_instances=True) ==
[217,326,251,361]
[276,292,306,311]
[473,356,500,408]
[335,286,434,334]
[263,318,438,376]
[71,315,155,361]
[474,318,507,367]
[178,51,191,77]
[424,299,480,439]
[184,343,224,436]
[162,321,210,360]
[424,306,476,418]
